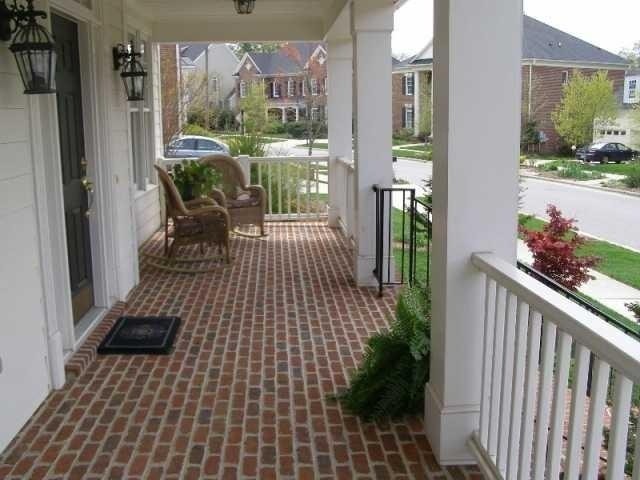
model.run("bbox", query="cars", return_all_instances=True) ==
[164,136,240,157]
[576,141,638,164]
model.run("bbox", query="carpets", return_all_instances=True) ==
[98,316,181,354]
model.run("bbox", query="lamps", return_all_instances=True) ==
[234,0,254,15]
[113,40,147,101]
[0,0,61,94]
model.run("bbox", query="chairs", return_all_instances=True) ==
[141,165,236,273]
[197,155,268,238]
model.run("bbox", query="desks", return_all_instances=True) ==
[166,192,221,255]
